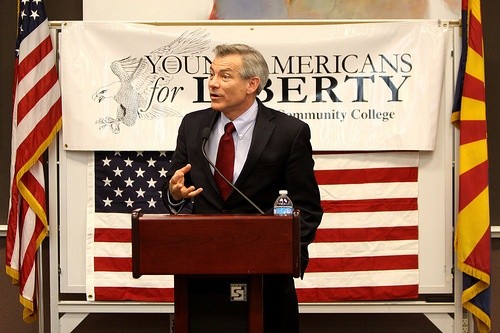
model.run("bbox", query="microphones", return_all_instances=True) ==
[201,127,266,215]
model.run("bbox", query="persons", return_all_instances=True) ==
[162,44,323,333]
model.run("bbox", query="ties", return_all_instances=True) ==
[214,122,236,201]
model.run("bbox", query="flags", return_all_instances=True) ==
[6,0,62,324]
[86,151,419,303]
[451,0,490,333]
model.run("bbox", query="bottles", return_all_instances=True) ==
[274,190,293,216]
